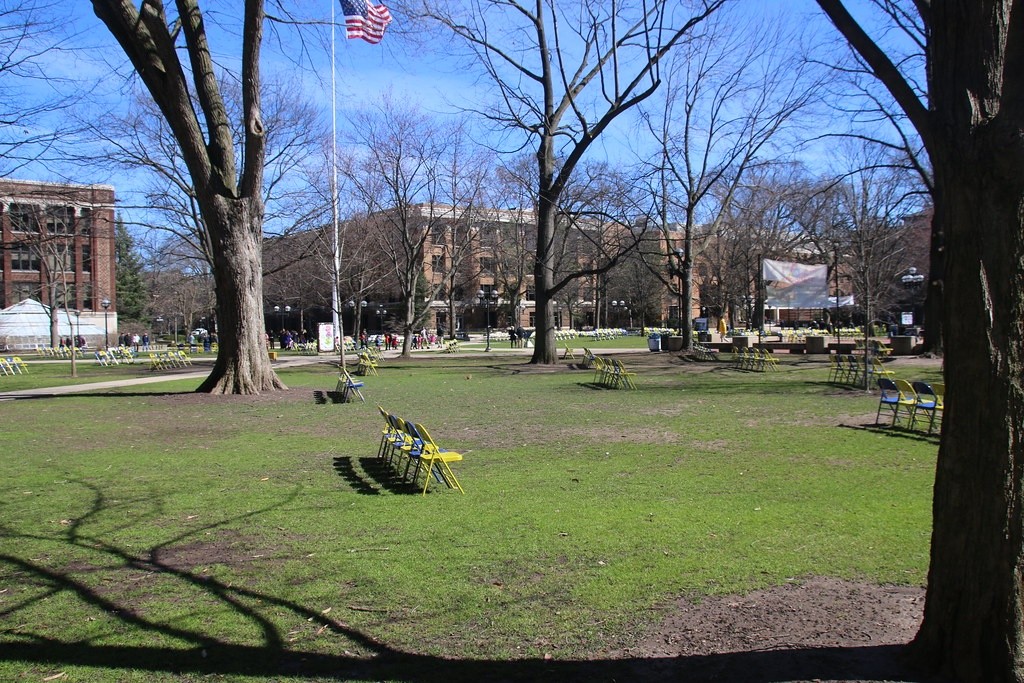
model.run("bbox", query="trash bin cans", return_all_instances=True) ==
[698,331,712,342]
[660,331,673,351]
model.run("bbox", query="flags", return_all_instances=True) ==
[339,0,392,44]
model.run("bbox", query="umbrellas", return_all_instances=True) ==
[0,298,106,350]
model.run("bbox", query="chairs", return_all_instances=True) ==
[293,337,385,404]
[376,405,464,496]
[94,346,140,367]
[445,338,462,353]
[149,342,219,371]
[37,345,83,359]
[484,330,511,342]
[529,326,945,436]
[0,356,30,377]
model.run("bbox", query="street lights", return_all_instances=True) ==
[901,267,924,328]
[516,305,524,328]
[74,310,81,344]
[612,301,627,329]
[476,290,497,351]
[376,310,387,333]
[275,306,290,331]
[675,247,681,336]
[834,241,841,356]
[100,298,110,350]
[349,301,367,336]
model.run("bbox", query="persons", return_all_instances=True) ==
[411,326,445,349]
[142,332,150,352]
[265,328,308,351]
[510,324,525,348]
[59,335,86,349]
[334,337,341,351]
[361,329,399,351]
[119,332,140,352]
[188,333,218,344]
[719,315,729,342]
